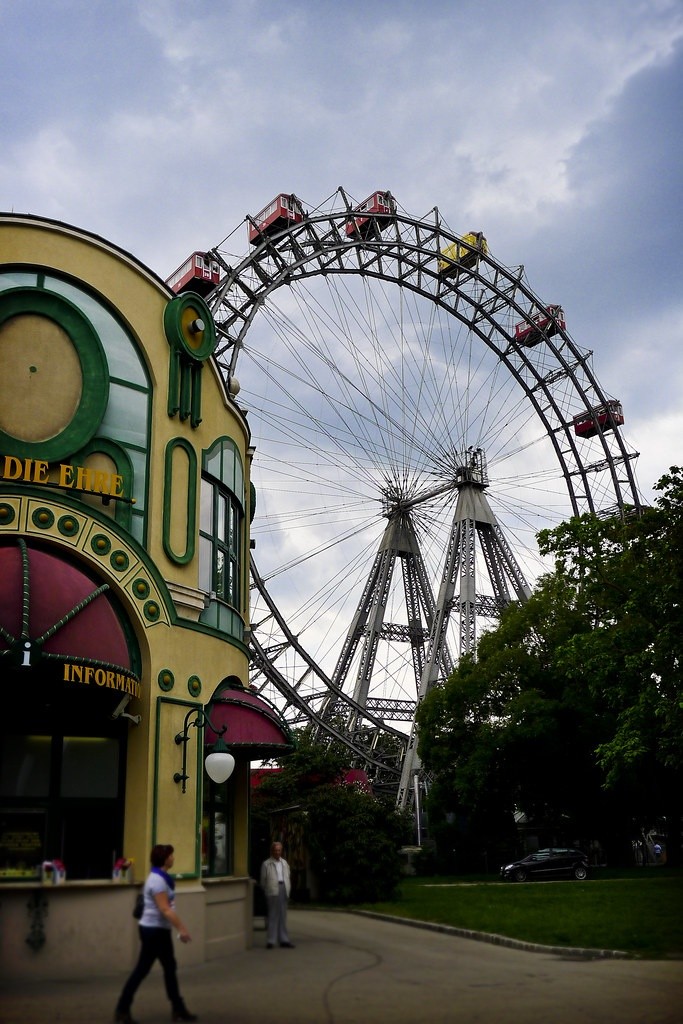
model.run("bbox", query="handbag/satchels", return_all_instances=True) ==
[131,894,145,919]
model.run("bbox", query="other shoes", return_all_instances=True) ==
[171,1006,197,1023]
[279,941,296,948]
[266,943,273,949]
[113,1009,140,1024]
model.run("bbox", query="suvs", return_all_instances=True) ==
[499,846,592,882]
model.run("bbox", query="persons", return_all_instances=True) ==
[654,842,663,862]
[115,844,197,1024]
[262,842,296,948]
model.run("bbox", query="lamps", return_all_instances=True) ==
[209,591,216,601]
[101,497,111,506]
[192,319,204,332]
[174,708,235,794]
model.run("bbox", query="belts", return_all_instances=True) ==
[278,881,284,884]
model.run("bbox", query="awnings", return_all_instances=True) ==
[1,537,142,699]
[207,686,297,762]
